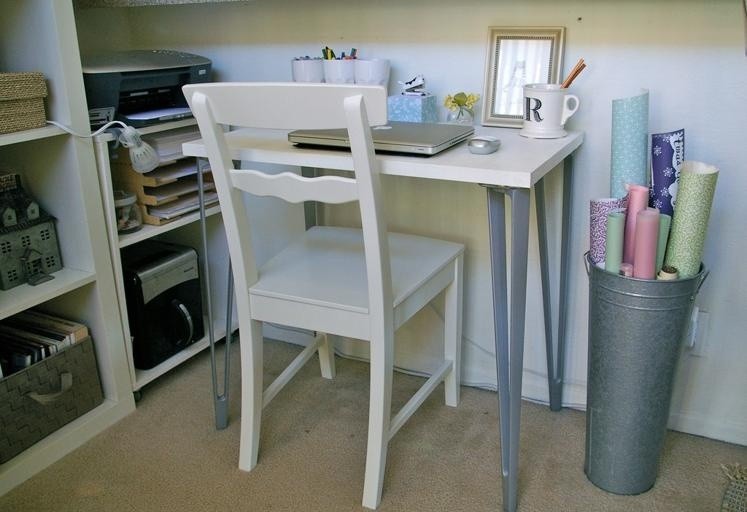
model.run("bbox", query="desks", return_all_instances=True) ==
[179,128,582,511]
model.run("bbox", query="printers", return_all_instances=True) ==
[81,47,212,129]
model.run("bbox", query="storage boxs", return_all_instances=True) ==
[1,314,102,468]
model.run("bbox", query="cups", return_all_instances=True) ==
[352,57,391,95]
[518,83,580,140]
[323,58,354,84]
[290,58,323,84]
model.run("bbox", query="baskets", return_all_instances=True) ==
[0,70,48,133]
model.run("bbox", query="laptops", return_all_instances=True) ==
[287,120,475,155]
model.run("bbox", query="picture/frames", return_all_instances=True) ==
[482,23,566,129]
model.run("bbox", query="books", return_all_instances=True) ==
[0,308,91,379]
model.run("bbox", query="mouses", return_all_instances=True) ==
[468,135,501,155]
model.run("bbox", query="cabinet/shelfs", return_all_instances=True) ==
[0,1,135,512]
[90,114,238,403]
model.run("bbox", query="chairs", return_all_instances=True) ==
[180,78,471,511]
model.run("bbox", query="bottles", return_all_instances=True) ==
[113,190,145,236]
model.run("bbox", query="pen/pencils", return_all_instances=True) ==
[559,58,586,89]
[322,46,357,60]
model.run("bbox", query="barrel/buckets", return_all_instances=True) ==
[582,250,711,495]
